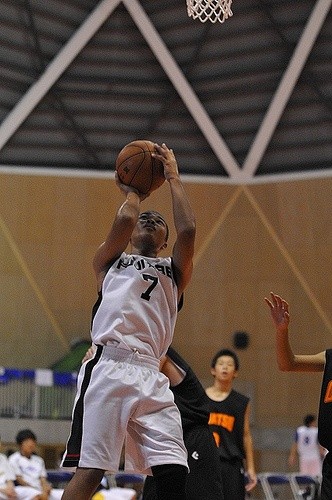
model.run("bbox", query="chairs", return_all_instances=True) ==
[46,471,145,500]
[245,472,322,500]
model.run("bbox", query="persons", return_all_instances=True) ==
[81,345,224,500]
[59,143,198,500]
[0,428,137,500]
[205,349,258,500]
[266,290,332,500]
[288,415,325,500]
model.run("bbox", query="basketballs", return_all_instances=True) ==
[115,140,171,195]
